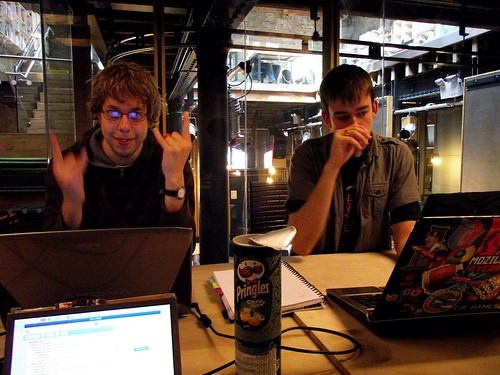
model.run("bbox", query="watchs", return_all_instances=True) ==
[160,186,186,199]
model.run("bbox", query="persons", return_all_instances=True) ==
[286,64,423,259]
[45,62,194,255]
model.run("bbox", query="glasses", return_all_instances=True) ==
[101,108,148,122]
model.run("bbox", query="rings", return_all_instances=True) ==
[344,130,348,135]
[162,134,170,139]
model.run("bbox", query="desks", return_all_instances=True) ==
[178,249,500,375]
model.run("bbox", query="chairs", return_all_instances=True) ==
[251,180,288,233]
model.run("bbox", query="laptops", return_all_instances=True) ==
[3,293,182,375]
[0,227,194,332]
[326,191,500,327]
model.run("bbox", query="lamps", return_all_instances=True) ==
[371,36,478,88]
